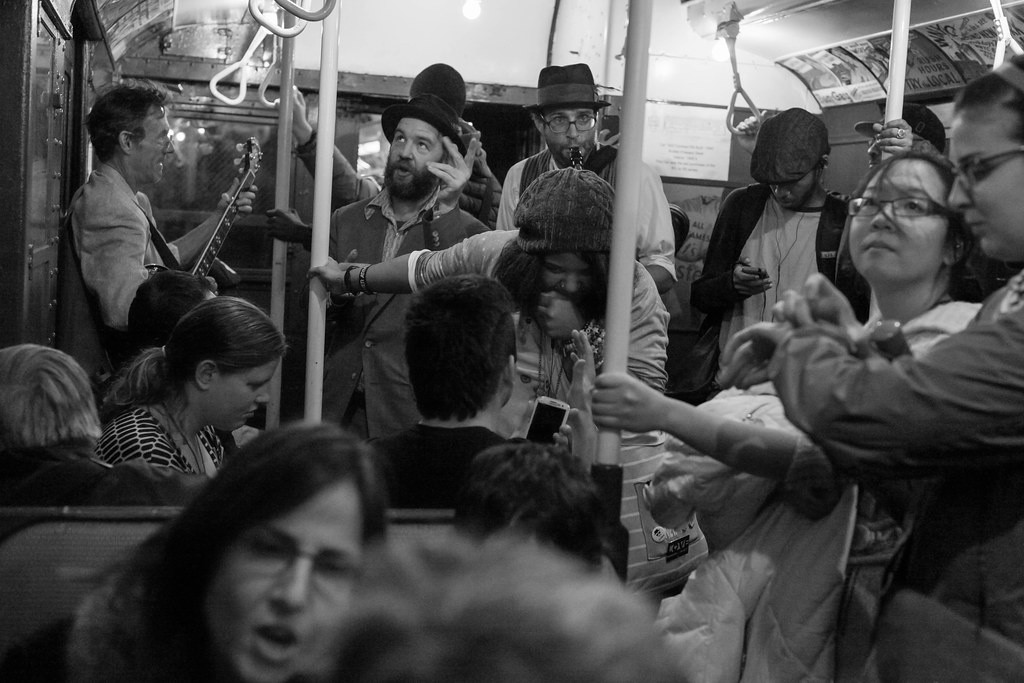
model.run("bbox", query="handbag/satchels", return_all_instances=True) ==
[833,561,1024,683]
[614,432,710,603]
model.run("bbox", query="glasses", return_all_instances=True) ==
[131,129,174,155]
[947,147,1024,191]
[231,530,362,609]
[868,134,923,149]
[845,196,951,217]
[539,113,596,134]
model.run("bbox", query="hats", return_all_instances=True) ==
[523,64,612,117]
[513,167,614,253]
[381,93,467,159]
[750,108,828,183]
[409,64,466,118]
[854,103,946,153]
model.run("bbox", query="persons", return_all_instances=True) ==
[0,54,1024,683]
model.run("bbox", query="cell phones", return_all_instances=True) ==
[741,267,761,275]
[526,396,570,445]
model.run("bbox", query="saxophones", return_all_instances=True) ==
[568,146,584,171]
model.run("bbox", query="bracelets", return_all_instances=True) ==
[358,263,377,296]
[344,265,360,297]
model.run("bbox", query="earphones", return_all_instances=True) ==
[819,161,825,169]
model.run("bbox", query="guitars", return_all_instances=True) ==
[103,136,265,379]
